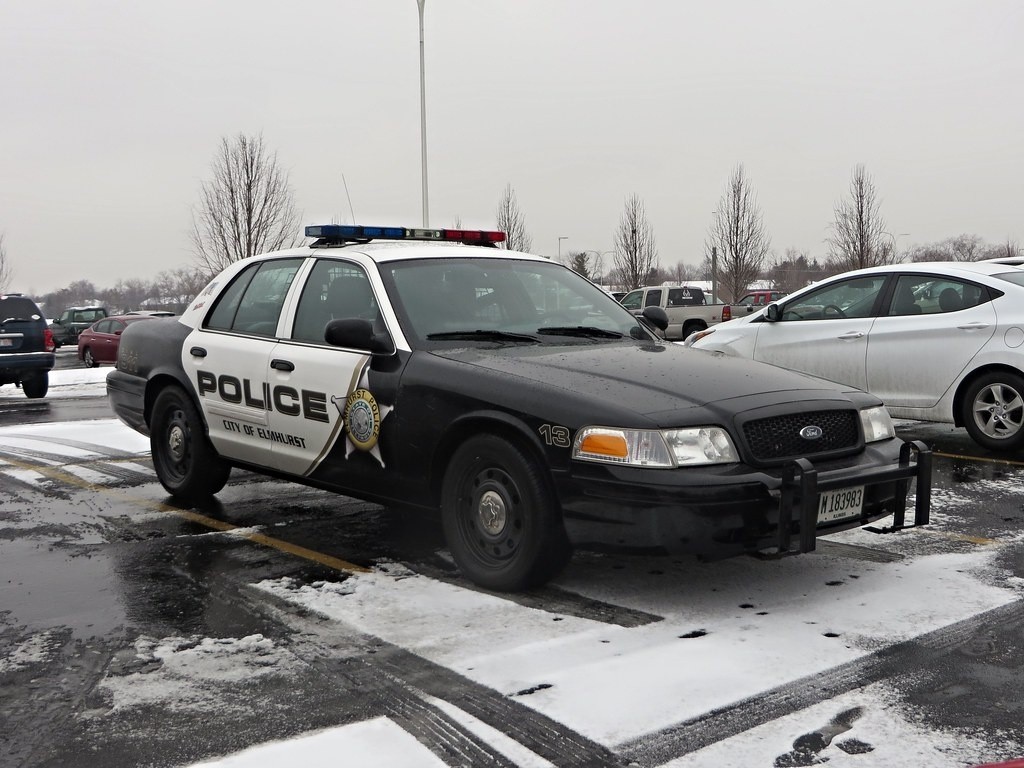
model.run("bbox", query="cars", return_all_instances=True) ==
[78,316,161,369]
[105,225,932,593]
[682,262,1024,452]
[124,311,177,317]
[604,291,625,304]
[700,292,724,305]
[910,256,1024,313]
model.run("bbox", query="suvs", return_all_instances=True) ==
[0,294,56,399]
[47,307,108,349]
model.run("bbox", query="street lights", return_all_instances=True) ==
[559,236,568,261]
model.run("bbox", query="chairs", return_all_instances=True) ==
[311,276,373,343]
[940,287,962,312]
[896,286,922,314]
[75,315,84,321]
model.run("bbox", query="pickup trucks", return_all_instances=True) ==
[620,285,731,342]
[728,291,831,321]
